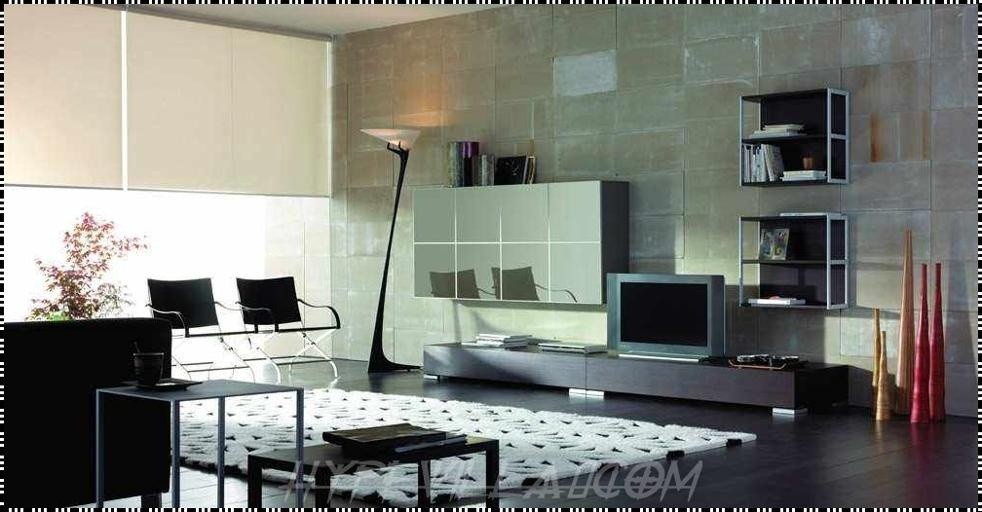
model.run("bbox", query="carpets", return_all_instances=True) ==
[171,387,758,510]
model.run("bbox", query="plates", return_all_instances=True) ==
[120,377,204,391]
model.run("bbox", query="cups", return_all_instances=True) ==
[132,352,164,386]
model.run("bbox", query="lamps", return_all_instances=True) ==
[358,128,422,374]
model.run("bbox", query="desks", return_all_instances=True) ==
[94,379,304,509]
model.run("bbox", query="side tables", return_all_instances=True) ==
[247,434,501,511]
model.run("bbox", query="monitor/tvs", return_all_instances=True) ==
[606,272,726,362]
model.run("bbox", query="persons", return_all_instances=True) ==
[759,232,772,260]
[777,231,787,250]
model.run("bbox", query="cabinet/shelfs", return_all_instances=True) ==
[411,179,629,305]
[737,88,849,311]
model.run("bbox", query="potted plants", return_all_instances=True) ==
[131,343,164,382]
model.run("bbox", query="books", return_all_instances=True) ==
[460,332,533,348]
[442,138,536,189]
[741,122,827,183]
[747,297,806,306]
[322,421,447,450]
[393,431,468,454]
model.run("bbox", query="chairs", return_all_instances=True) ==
[145,276,342,380]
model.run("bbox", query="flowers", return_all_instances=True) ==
[22,211,150,321]
